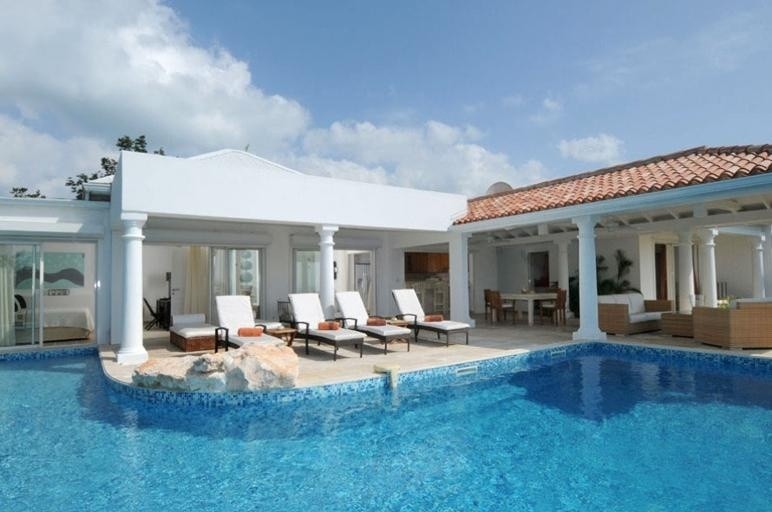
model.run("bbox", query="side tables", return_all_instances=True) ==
[266,328,298,347]
[386,319,408,344]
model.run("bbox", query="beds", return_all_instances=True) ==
[13,294,95,344]
[170,313,219,353]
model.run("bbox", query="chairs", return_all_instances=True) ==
[483,288,568,328]
[334,291,411,355]
[215,295,283,353]
[144,296,171,331]
[287,293,364,362]
[392,289,471,347]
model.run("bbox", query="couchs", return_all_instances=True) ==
[693,297,771,350]
[598,293,675,337]
